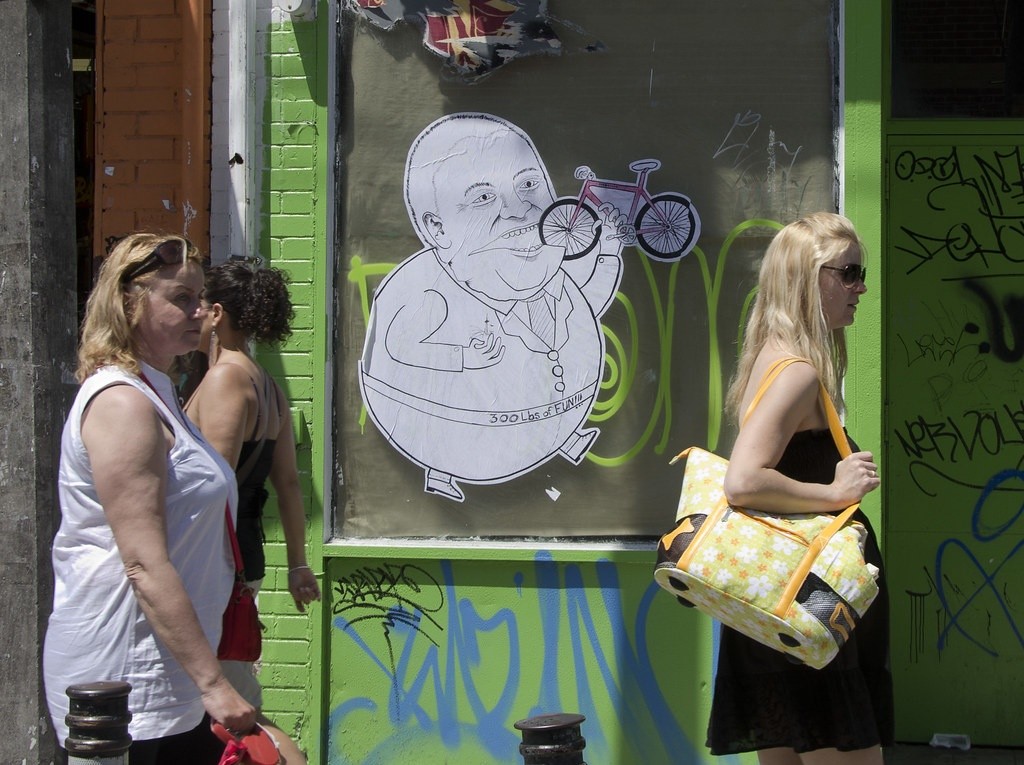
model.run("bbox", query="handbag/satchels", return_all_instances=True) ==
[216,580,262,661]
[655,357,878,670]
[211,717,282,765]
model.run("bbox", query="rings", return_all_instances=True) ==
[873,471,876,478]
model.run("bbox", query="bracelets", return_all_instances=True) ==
[287,566,311,574]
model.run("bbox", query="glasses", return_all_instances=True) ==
[124,239,193,282]
[818,263,867,288]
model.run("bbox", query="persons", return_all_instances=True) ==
[705,211,894,765]
[43,233,257,765]
[182,255,320,715]
